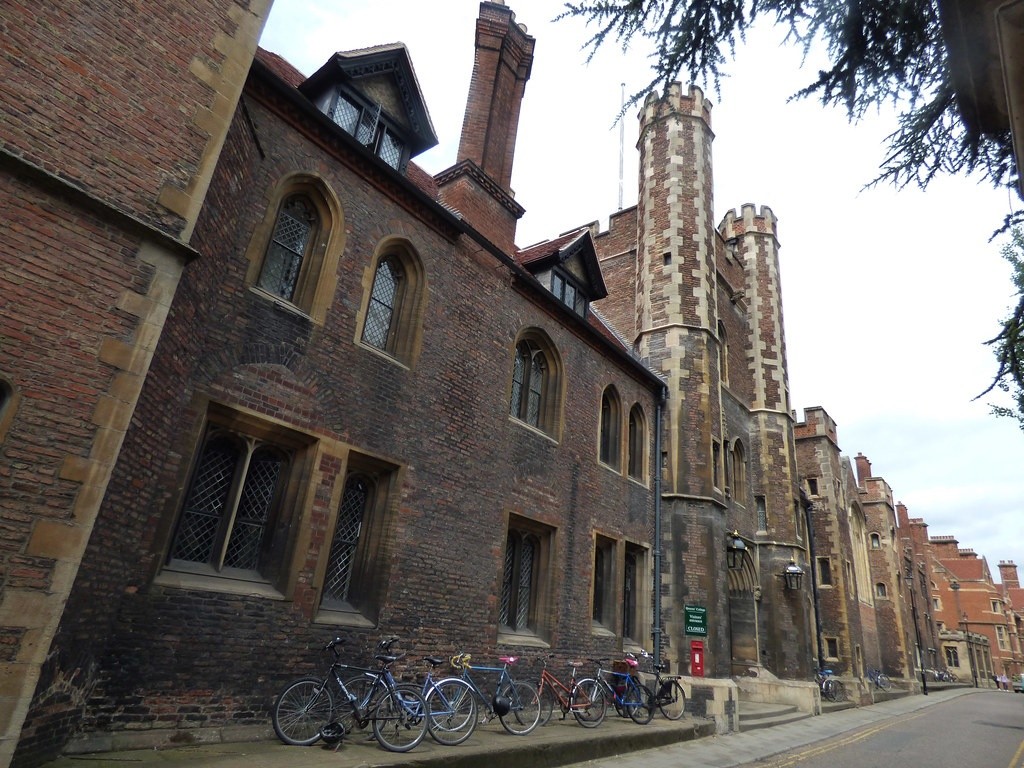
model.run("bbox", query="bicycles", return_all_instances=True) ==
[615,655,688,720]
[866,666,892,693]
[513,650,608,729]
[574,658,657,725]
[333,635,479,747]
[932,666,961,682]
[816,669,845,703]
[270,635,432,753]
[427,651,542,736]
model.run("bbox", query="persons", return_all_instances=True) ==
[1000,672,1009,691]
[994,672,1001,690]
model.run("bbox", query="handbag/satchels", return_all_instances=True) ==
[617,684,629,692]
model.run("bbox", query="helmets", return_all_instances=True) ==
[493,694,509,716]
[319,722,346,744]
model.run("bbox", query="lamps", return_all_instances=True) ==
[774,556,805,590]
[727,530,746,570]
